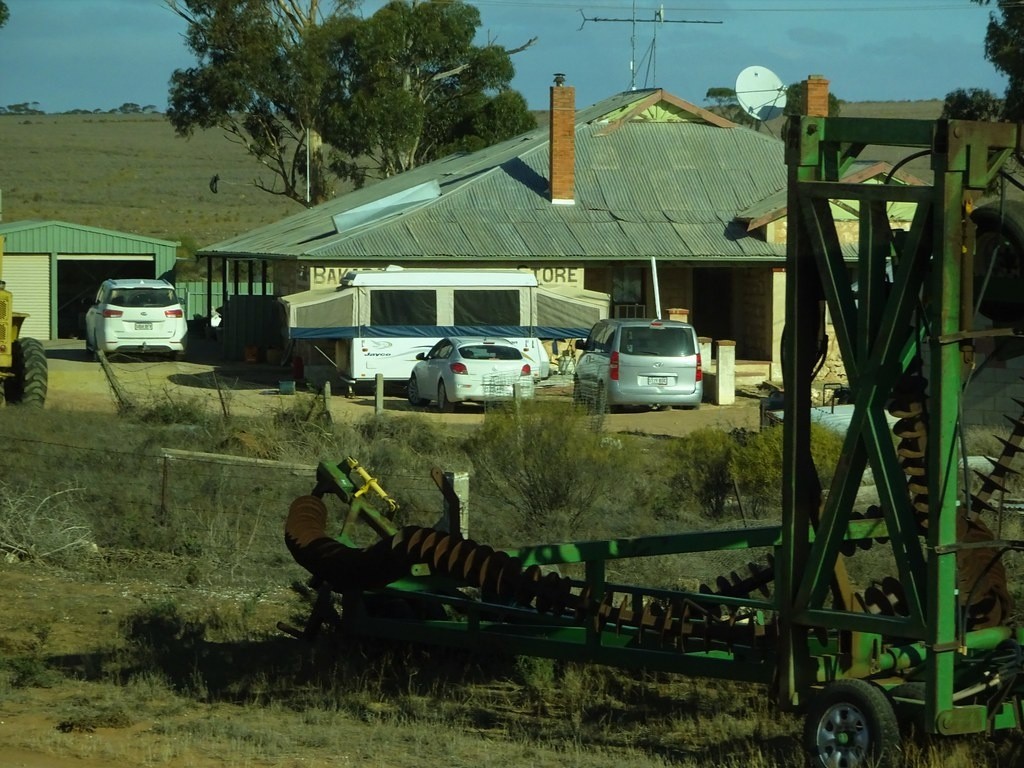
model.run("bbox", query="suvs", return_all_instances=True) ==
[84,277,188,364]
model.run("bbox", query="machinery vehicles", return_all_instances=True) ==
[1,231,48,411]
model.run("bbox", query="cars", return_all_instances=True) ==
[406,335,534,412]
[571,314,703,413]
